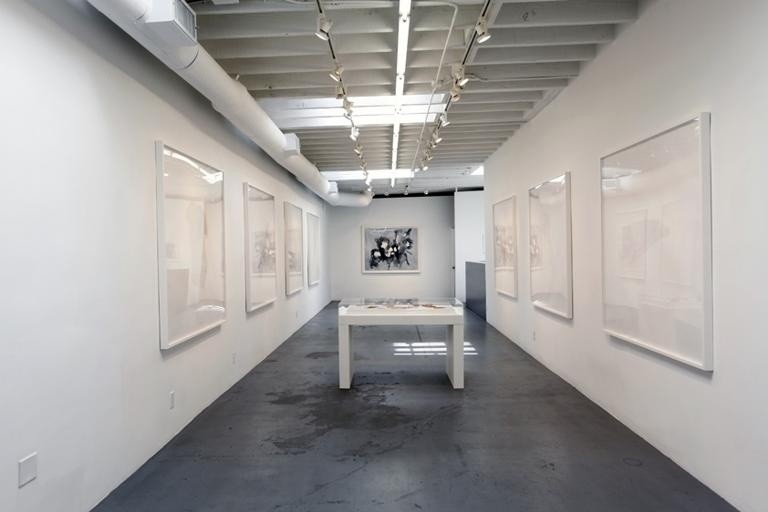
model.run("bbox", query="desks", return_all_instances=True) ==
[336,301,467,391]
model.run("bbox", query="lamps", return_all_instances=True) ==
[311,1,492,199]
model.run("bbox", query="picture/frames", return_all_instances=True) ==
[306,210,321,287]
[282,198,305,303]
[156,135,227,354]
[491,194,518,300]
[600,111,713,379]
[527,170,572,323]
[361,225,420,276]
[242,181,277,314]
